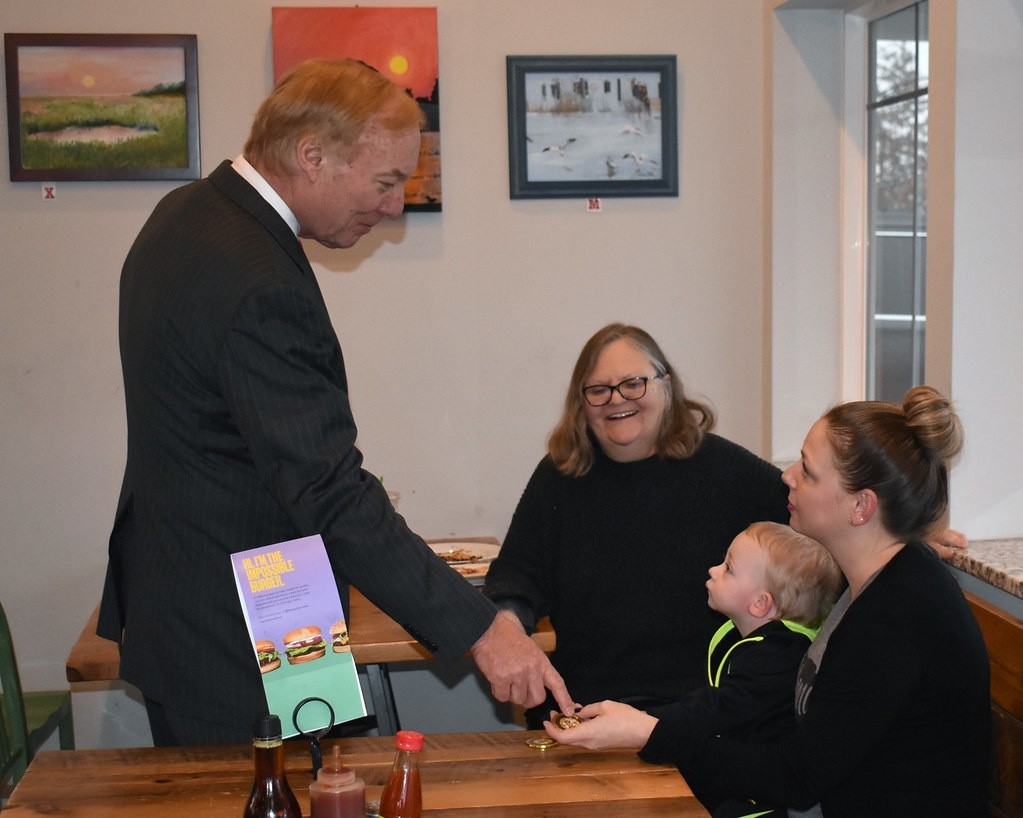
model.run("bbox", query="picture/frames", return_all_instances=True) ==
[3,31,202,184]
[505,54,680,199]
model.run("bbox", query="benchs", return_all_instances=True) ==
[961,589,1023,818]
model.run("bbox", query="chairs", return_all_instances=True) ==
[0,603,75,786]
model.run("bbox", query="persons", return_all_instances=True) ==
[481,322,970,733]
[637,518,842,805]
[543,382,992,818]
[94,54,576,749]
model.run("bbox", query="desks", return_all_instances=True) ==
[0,731,710,818]
[67,535,557,737]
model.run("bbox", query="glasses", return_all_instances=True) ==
[582,370,667,408]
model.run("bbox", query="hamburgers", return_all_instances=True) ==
[283,624,326,665]
[329,619,351,653]
[255,640,281,673]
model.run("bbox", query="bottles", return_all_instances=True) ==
[378,730,424,818]
[309,745,366,818]
[244,715,302,818]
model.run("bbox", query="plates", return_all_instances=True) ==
[427,542,501,564]
[448,564,490,585]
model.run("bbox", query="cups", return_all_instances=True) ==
[387,491,401,510]
